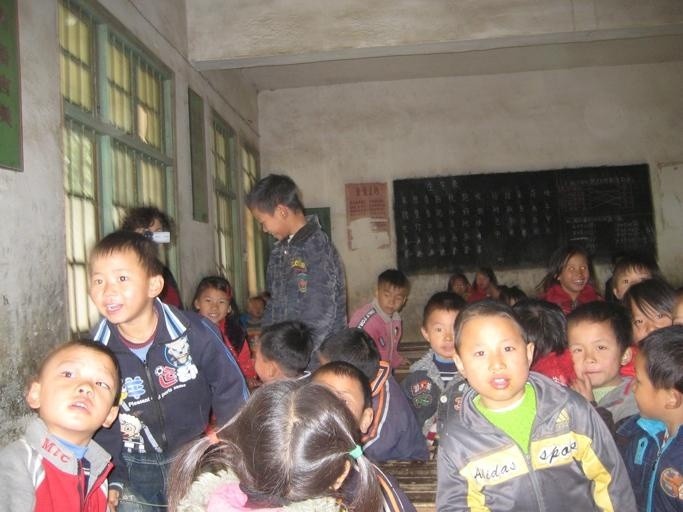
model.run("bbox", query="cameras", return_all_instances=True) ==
[145,230,171,245]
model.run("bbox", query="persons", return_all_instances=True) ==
[241,171,351,382]
[83,230,256,512]
[120,205,185,314]
[404,243,683,511]
[1,267,431,511]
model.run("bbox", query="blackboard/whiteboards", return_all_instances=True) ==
[394,163,661,275]
